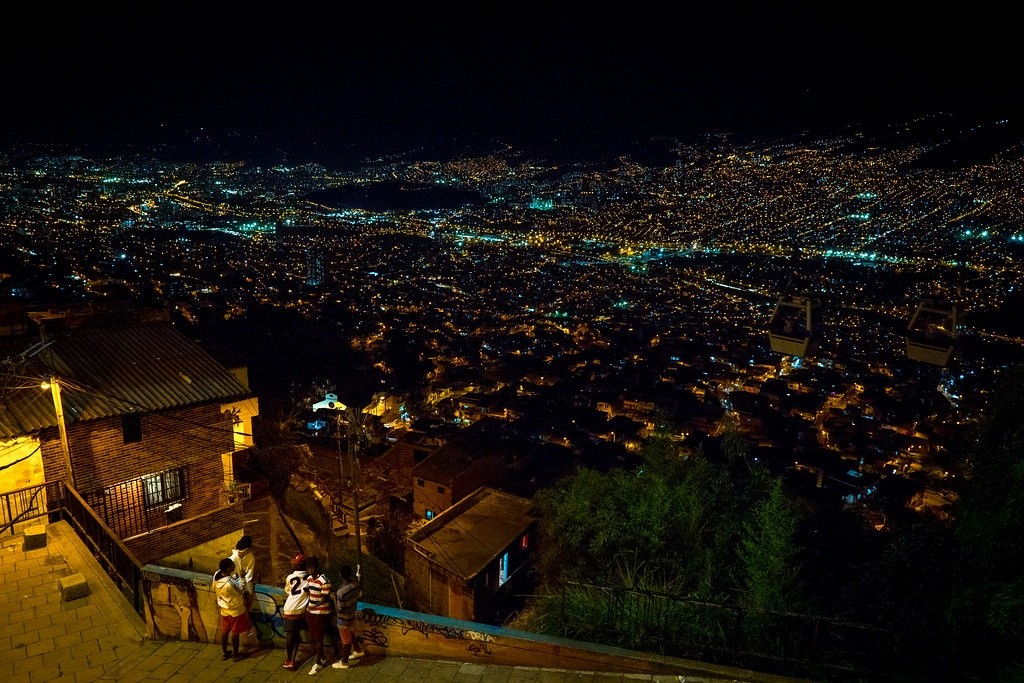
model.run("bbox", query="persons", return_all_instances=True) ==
[212,536,255,662]
[283,555,365,675]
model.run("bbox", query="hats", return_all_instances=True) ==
[290,554,307,564]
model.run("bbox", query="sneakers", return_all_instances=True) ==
[348,650,365,660]
[283,659,293,668]
[320,659,326,664]
[308,663,323,675]
[331,659,350,669]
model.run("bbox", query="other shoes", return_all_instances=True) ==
[221,649,247,662]
